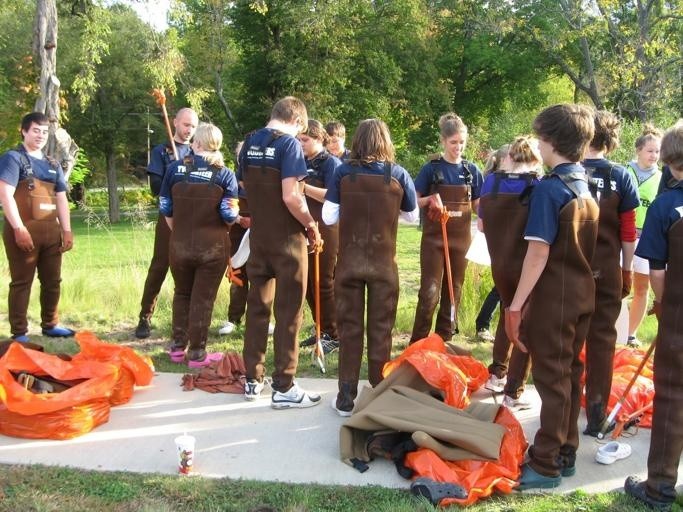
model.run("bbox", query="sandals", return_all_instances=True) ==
[410,477,468,506]
[595,441,632,464]
[512,444,575,490]
[169,350,224,368]
[625,475,673,512]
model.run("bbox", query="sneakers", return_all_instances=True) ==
[484,373,532,412]
[478,327,495,342]
[218,321,237,336]
[301,327,339,355]
[267,322,274,335]
[244,379,264,401]
[271,385,321,410]
[331,397,352,417]
[136,319,151,338]
[42,325,75,338]
[12,335,30,342]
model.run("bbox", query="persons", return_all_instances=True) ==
[320,119,416,416]
[0,112,74,344]
[135,107,240,369]
[219,95,352,409]
[624,121,682,507]
[475,103,665,490]
[407,113,485,348]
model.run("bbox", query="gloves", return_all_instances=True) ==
[427,193,443,222]
[240,217,250,229]
[13,225,35,253]
[57,231,73,253]
[504,306,529,353]
[307,221,320,255]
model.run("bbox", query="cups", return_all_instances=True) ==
[175,435,195,474]
